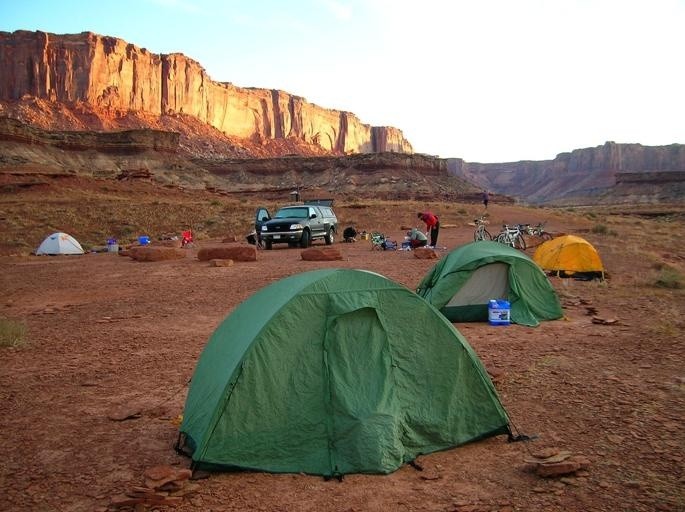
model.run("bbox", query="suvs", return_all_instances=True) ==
[255,200,338,248]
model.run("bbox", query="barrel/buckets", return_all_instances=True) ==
[107,239,119,252]
[138,236,152,244]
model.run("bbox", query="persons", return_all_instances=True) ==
[481,190,489,209]
[410,227,427,249]
[416,210,440,247]
[343,226,357,243]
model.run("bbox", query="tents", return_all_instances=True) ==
[532,235,608,283]
[416,242,564,329]
[173,267,522,481]
[35,232,85,257]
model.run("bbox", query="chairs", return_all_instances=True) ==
[246,233,263,254]
[370,232,397,251]
[180,228,197,249]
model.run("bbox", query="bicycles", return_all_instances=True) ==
[473,213,552,250]
